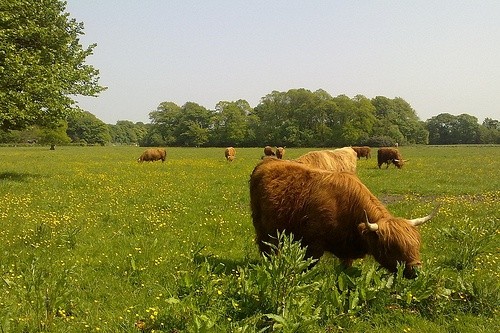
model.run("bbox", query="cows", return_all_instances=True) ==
[249,158,432,280]
[350,146,372,160]
[225,147,236,162]
[263,146,284,159]
[292,146,357,174]
[137,148,166,163]
[377,147,409,169]
